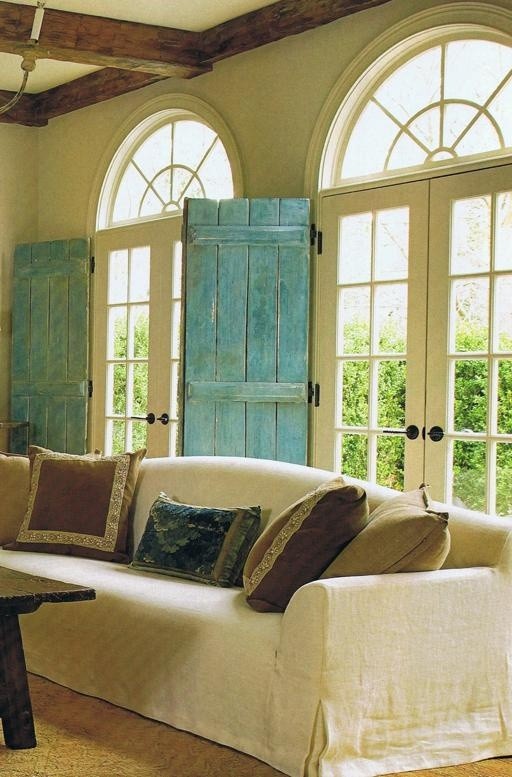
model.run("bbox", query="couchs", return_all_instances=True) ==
[0,448,511,776]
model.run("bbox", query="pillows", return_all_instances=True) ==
[131,490,264,584]
[318,481,452,580]
[15,441,147,565]
[240,471,371,611]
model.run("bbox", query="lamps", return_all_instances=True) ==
[0,0,49,115]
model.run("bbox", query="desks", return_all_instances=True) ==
[0,565,100,751]
